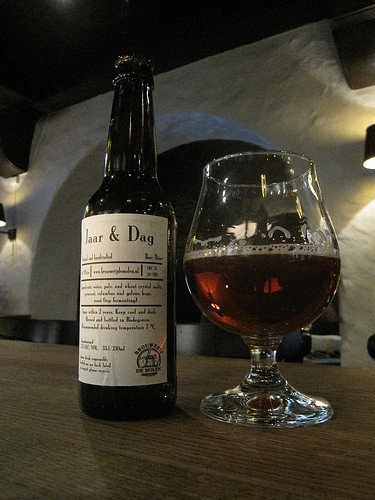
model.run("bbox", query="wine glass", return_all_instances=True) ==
[184,151,342,430]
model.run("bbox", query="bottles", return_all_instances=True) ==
[78,54,178,422]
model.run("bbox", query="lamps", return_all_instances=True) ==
[362,123,375,170]
[0,202,17,241]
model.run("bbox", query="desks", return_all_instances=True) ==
[0,341,375,500]
